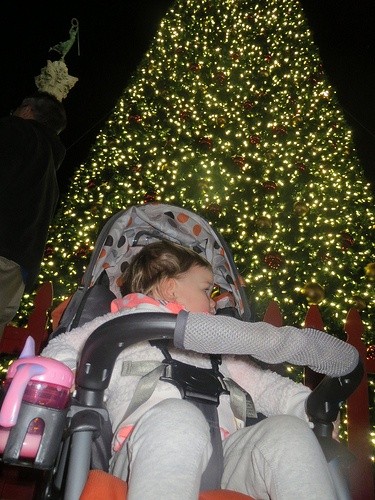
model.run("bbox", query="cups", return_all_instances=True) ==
[0,336,76,470]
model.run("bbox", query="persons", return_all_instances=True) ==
[0,91,67,340]
[38,240,340,500]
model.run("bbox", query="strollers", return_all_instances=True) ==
[0,201,365,500]
